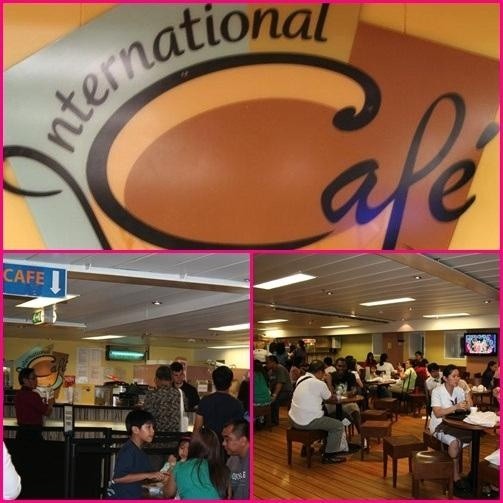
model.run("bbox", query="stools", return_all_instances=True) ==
[253,370,499,498]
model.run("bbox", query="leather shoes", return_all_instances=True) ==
[301,445,314,457]
[321,454,346,463]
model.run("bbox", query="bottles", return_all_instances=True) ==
[335,390,341,399]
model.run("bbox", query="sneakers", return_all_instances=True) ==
[453,480,471,498]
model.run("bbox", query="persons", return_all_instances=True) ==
[13,367,54,500]
[254,333,500,464]
[427,364,471,493]
[103,362,248,498]
[2,442,20,498]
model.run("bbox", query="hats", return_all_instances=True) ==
[447,409,469,419]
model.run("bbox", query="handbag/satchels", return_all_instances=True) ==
[463,411,497,427]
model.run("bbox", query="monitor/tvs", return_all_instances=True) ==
[464,333,498,356]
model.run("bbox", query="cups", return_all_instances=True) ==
[65,385,75,404]
[469,406,478,412]
